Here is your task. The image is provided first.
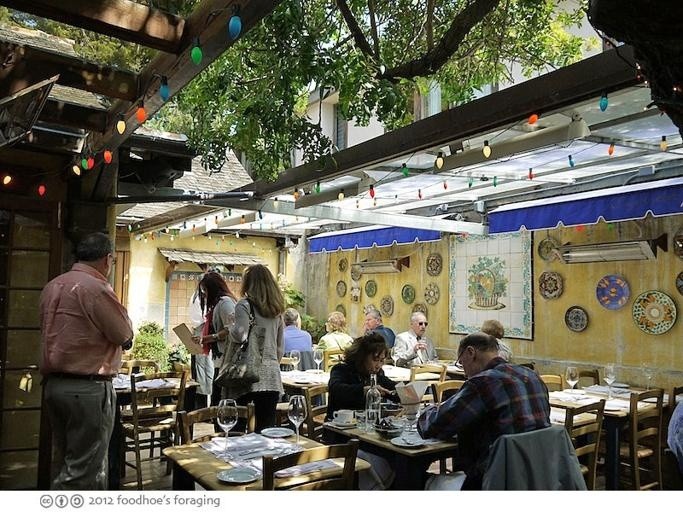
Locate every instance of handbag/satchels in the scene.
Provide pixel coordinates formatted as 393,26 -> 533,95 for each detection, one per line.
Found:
214,322 -> 266,385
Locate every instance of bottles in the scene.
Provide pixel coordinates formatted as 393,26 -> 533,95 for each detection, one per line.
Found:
366,374 -> 381,433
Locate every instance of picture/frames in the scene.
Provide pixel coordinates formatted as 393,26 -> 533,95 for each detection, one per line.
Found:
448,231 -> 535,341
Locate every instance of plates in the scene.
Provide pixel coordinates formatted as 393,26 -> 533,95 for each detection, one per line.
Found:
538,238 -> 559,261
596,274 -> 630,310
676,271 -> 683,295
351,267 -> 361,280
217,469 -> 260,483
391,437 -> 422,447
468,302 -> 505,309
336,304 -> 345,316
565,306 -> 588,332
351,288 -> 361,303
261,427 -> 294,438
632,290 -> 677,335
401,284 -> 415,304
336,281 -> 346,297
674,226 -> 683,258
605,407 -> 621,411
365,280 -> 377,297
538,272 -> 563,298
338,258 -> 348,272
564,389 -> 585,395
612,383 -> 629,387
332,422 -> 357,426
644,394 -> 680,402
295,381 -> 310,384
425,283 -> 440,304
381,296 -> 393,316
426,254 -> 441,275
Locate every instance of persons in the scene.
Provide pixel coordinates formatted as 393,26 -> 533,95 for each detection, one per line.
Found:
38,232 -> 134,490
220,264 -> 285,433
417,332 -> 552,490
283,308 -> 312,353
191,272 -> 238,406
482,320 -> 513,363
322,332 -> 402,490
363,308 -> 395,350
317,311 -> 354,372
667,399 -> 683,471
189,265 -> 230,397
391,311 -> 436,368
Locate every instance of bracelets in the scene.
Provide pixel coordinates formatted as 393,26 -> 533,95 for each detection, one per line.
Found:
212,334 -> 218,342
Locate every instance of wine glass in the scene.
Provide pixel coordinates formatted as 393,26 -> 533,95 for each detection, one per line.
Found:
313,348 -> 324,374
565,367 -> 578,394
288,395 -> 307,451
391,347 -> 399,368
290,350 -> 300,372
604,360 -> 617,400
216,399 -> 238,459
641,360 -> 654,391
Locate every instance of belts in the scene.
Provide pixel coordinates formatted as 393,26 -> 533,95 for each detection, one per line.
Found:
39,373 -> 112,383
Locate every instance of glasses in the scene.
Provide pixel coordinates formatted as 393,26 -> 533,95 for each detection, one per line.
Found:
454,346 -> 467,368
109,254 -> 117,265
418,321 -> 428,326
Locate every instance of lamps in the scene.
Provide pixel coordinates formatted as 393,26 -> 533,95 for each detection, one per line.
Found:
295,170 -> 378,209
180,218 -> 212,238
433,108 -> 592,176
551,234 -> 668,265
217,210 -> 261,229
351,256 -> 410,274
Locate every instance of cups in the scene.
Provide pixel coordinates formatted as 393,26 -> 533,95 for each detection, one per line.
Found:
333,409 -> 353,424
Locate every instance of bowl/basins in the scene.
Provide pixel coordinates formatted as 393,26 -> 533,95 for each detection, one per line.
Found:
380,403 -> 403,416
375,424 -> 403,438
